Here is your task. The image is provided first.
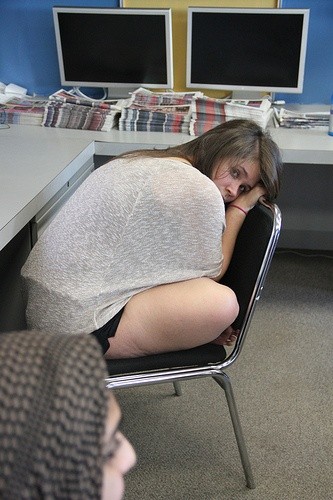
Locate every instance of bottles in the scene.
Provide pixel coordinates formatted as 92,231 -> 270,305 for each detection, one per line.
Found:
329,96 -> 333,136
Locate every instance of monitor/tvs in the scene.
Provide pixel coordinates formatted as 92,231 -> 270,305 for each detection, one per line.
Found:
52,7 -> 173,98
186,7 -> 310,100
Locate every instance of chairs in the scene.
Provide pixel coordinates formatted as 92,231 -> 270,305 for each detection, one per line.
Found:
106,197 -> 282,489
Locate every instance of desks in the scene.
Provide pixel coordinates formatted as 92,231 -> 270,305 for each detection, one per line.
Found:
0,122 -> 94,251
94,101 -> 333,253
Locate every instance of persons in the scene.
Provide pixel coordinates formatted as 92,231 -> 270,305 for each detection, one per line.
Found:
0,330 -> 137,500
21,118 -> 284,360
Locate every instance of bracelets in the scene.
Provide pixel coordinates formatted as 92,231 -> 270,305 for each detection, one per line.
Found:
228,203 -> 249,216
